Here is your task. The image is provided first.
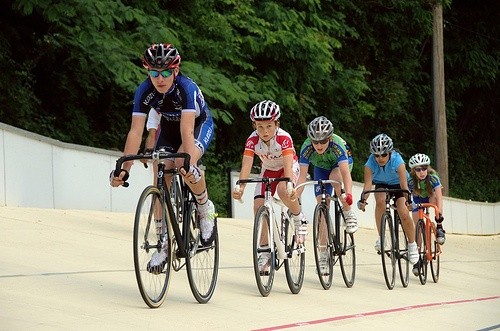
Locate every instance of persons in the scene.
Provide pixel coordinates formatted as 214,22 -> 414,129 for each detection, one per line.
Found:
288,117 -> 358,272
409,153 -> 445,275
233,100 -> 309,269
110,43 -> 214,267
358,133 -> 419,264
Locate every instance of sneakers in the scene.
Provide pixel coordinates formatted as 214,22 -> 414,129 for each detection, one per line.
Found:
407,242 -> 419,264
344,211 -> 358,233
294,215 -> 308,244
198,200 -> 215,246
146,234 -> 168,274
413,259 -> 422,274
436,229 -> 445,244
375,237 -> 387,251
317,254 -> 329,275
258,253 -> 272,272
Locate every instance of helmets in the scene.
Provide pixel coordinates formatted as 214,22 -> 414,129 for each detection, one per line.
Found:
142,43 -> 181,70
307,116 -> 334,141
249,100 -> 282,122
370,133 -> 393,154
409,153 -> 430,168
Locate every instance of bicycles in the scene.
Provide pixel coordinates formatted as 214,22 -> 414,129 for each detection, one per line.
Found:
360,188 -> 411,290
411,203 -> 443,285
114,148 -> 219,309
295,179 -> 357,291
234,177 -> 306,298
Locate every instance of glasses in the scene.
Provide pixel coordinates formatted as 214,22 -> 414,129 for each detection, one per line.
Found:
311,138 -> 330,144
415,168 -> 427,172
375,151 -> 388,157
147,67 -> 175,78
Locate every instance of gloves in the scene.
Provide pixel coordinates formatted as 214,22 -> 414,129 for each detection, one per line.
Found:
435,213 -> 444,222
